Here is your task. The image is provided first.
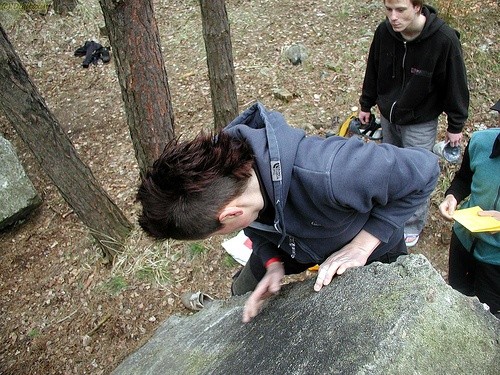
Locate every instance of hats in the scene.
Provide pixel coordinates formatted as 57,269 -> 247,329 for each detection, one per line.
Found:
490,98 -> 500,111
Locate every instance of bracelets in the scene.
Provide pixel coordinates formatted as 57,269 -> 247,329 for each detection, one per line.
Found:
266,257 -> 278,267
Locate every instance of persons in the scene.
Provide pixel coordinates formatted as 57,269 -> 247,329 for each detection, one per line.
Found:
135,104 -> 439,324
359,0 -> 469,246
439,99 -> 500,317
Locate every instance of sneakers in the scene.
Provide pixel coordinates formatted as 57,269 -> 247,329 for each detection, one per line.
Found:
181,291 -> 215,311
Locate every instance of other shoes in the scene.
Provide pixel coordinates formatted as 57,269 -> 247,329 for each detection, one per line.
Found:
349,113 -> 383,140
404,232 -> 419,246
